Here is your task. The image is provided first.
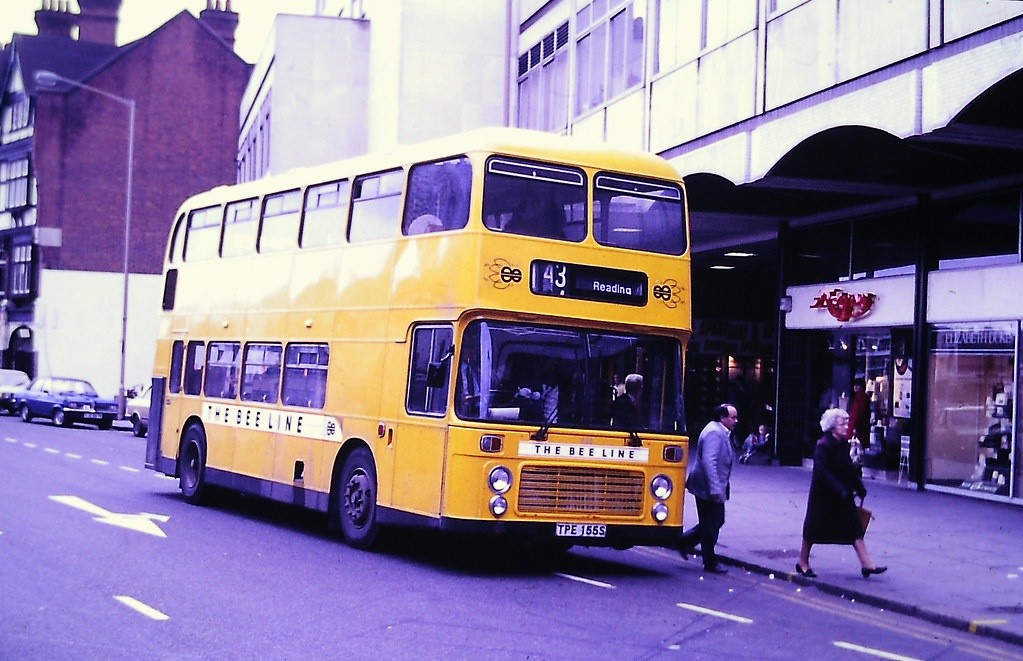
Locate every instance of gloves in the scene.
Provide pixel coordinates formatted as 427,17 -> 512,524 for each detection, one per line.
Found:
856,487 -> 866,499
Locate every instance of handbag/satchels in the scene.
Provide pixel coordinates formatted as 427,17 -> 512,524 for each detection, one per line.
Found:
853,493 -> 872,539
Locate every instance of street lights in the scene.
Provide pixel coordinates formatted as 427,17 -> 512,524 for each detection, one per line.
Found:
33,70 -> 137,423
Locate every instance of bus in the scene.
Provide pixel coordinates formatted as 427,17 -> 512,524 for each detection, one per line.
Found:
144,127 -> 693,563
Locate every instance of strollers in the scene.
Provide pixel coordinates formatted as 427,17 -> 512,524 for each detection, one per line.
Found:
737,433 -> 770,464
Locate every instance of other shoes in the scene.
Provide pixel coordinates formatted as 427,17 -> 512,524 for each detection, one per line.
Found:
703,562 -> 729,573
679,548 -> 688,560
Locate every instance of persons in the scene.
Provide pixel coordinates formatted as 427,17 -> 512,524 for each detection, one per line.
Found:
795,408 -> 887,580
844,378 -> 876,480
739,424 -> 771,465
678,402 -> 739,573
440,338 -> 648,429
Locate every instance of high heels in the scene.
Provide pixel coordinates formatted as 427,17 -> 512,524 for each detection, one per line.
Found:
796,563 -> 817,578
861,565 -> 887,578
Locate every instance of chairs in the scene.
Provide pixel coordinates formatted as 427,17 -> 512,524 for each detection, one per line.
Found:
747,433 -> 774,466
198,365 -> 237,399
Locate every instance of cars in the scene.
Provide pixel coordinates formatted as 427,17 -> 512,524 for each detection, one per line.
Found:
126,385 -> 154,438
0,368 -> 31,415
11,376 -> 120,431
